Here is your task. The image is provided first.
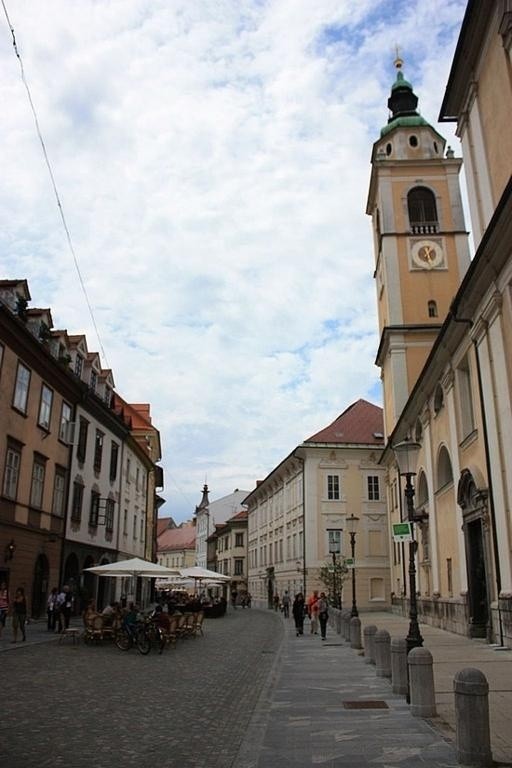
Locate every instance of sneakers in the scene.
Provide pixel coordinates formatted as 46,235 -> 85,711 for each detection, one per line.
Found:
296,628 -> 326,640
10,628 -> 69,643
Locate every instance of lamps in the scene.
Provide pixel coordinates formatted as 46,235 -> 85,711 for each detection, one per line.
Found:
3,535 -> 16,563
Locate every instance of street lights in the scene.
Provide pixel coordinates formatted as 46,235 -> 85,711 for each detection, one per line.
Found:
387,431 -> 427,706
327,537 -> 342,609
343,511 -> 361,617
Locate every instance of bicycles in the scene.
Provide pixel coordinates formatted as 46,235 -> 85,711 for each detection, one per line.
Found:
113,609 -> 153,655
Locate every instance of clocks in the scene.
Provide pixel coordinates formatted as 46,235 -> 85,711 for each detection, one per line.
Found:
406,236 -> 449,271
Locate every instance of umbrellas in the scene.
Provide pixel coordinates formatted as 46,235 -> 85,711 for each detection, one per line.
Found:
82,556 -> 232,611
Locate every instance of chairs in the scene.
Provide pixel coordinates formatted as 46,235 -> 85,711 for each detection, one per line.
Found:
61,596 -> 229,653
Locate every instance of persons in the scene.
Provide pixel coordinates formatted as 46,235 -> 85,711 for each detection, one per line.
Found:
273,590 -> 330,640
0,579 -> 227,646
232,588 -> 252,610
